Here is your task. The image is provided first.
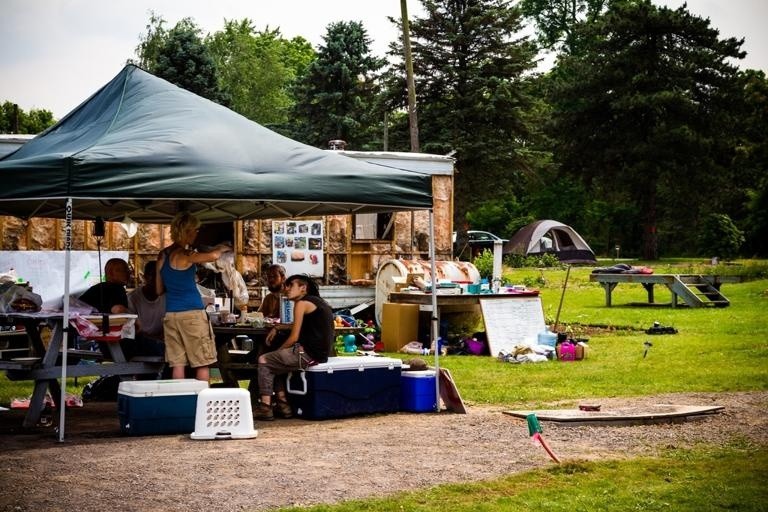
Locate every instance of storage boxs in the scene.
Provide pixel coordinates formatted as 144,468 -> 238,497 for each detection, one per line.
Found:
282,355 -> 441,422
115,377 -> 212,435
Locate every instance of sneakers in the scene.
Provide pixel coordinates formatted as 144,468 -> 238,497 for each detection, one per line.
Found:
252,405 -> 274,421
273,402 -> 294,419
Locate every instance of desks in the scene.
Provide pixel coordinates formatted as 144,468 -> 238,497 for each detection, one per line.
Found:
0,309 -> 140,430
387,283 -> 543,356
207,320 -> 365,403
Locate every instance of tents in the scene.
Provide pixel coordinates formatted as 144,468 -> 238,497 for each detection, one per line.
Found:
501,217 -> 599,269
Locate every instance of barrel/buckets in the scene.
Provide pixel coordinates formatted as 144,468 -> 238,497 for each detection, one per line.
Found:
374,261 -> 485,330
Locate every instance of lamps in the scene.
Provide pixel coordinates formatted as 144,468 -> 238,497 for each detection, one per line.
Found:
89,215 -> 108,244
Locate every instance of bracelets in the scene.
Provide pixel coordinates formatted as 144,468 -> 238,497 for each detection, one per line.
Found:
274,326 -> 279,332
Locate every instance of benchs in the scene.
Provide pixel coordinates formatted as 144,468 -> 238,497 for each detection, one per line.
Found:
0,347 -> 268,428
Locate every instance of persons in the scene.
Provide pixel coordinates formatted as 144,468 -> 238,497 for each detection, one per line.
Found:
156,211 -> 235,384
126,261 -> 165,354
75,257 -> 154,353
455,218 -> 472,260
243,271 -> 336,420
234,264 -> 288,385
